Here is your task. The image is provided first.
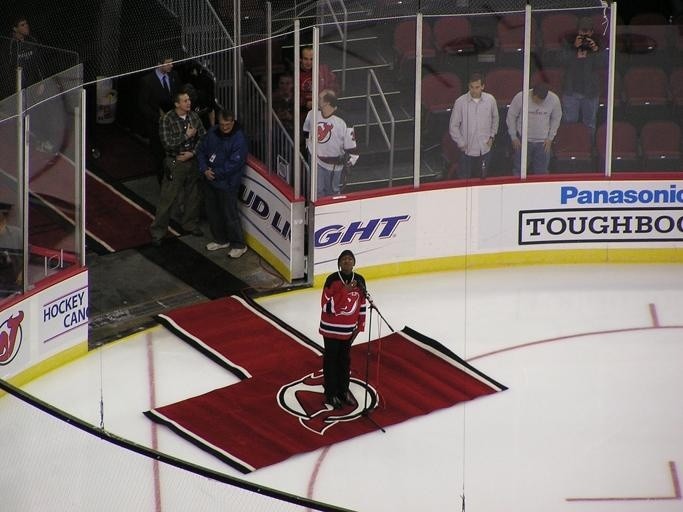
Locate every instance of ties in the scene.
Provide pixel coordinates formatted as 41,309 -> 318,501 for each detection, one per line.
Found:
161,74 -> 170,96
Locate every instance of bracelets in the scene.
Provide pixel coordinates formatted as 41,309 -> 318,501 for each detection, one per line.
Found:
489,137 -> 494,140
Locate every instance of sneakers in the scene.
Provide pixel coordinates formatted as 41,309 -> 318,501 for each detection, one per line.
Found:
324,394 -> 344,409
338,392 -> 356,406
205,240 -> 229,252
228,247 -> 248,258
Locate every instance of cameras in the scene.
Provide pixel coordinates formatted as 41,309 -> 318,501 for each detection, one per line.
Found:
578,35 -> 591,48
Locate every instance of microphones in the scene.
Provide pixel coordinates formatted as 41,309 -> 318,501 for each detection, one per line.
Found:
357,280 -> 366,291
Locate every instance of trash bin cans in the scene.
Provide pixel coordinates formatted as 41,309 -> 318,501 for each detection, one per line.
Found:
96,89 -> 118,125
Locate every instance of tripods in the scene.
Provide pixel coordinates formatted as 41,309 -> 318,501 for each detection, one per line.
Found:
344,291 -> 395,432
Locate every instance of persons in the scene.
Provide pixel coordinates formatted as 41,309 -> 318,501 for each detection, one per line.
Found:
504,83 -> 563,176
301,89 -> 360,199
447,70 -> 500,178
1,13 -> 43,97
196,106 -> 251,260
139,50 -> 182,188
557,16 -> 605,146
266,70 -> 308,138
291,44 -> 341,113
147,89 -> 206,249
317,247 -> 367,410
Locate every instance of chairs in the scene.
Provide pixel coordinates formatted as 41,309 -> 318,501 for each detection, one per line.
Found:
390,0 -> 683,179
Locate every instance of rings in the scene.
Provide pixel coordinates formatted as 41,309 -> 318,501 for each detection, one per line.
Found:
351,284 -> 353,286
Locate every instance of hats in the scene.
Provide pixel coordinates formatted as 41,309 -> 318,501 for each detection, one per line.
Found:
338,249 -> 356,264
